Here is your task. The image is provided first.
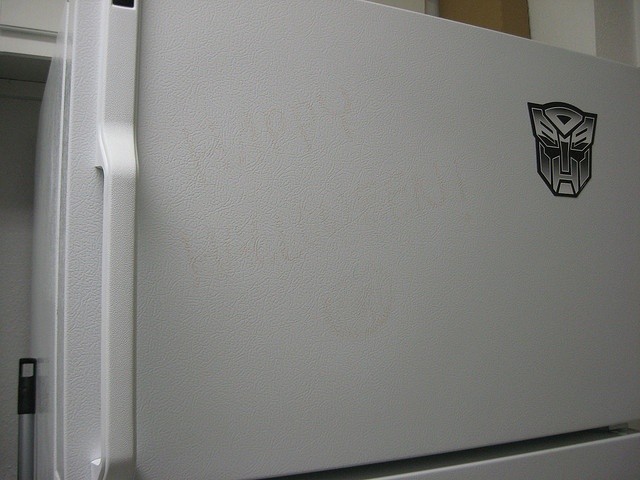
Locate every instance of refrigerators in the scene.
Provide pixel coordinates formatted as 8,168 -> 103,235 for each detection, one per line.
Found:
1,1 -> 640,479
